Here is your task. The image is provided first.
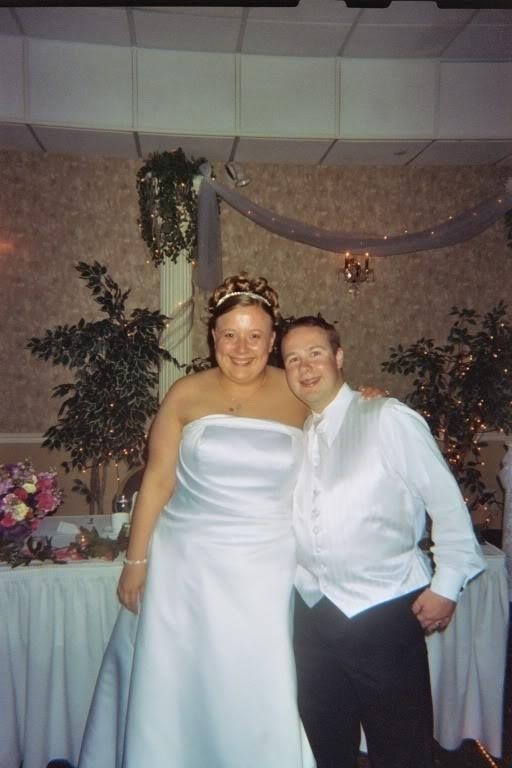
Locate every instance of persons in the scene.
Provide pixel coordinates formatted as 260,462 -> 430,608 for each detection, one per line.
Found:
74,276 -> 391,768
277,316 -> 486,767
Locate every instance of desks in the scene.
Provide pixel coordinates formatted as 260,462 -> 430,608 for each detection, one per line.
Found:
0,530 -> 510,768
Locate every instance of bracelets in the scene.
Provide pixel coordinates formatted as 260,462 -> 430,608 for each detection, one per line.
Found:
124,557 -> 147,565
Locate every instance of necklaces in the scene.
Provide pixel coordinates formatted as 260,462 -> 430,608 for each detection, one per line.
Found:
223,375 -> 267,411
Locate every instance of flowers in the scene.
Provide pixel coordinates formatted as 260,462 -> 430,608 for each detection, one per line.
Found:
0,457 -> 69,564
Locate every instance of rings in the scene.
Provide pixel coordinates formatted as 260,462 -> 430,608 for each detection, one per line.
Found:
385,390 -> 390,395
435,622 -> 441,628
